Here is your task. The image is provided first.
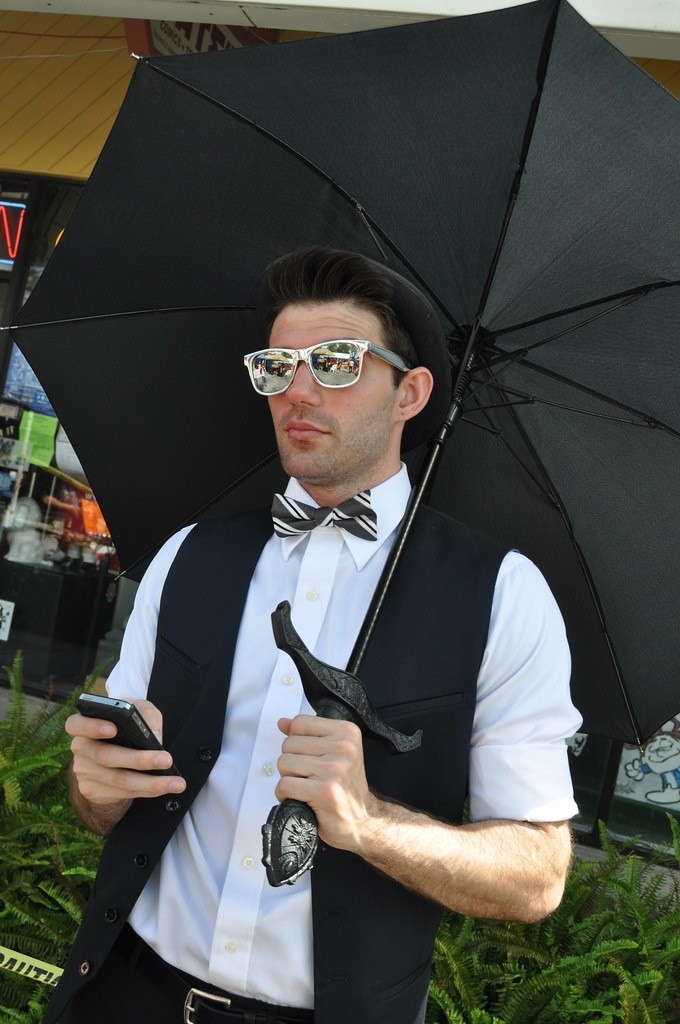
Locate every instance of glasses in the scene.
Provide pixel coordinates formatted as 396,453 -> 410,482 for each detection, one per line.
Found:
243,340 -> 412,396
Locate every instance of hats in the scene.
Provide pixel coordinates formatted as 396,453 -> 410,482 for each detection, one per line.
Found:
257,246 -> 451,454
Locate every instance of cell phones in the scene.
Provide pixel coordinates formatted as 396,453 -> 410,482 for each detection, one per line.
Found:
75,693 -> 180,780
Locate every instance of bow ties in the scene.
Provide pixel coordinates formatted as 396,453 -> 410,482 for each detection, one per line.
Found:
270,489 -> 376,543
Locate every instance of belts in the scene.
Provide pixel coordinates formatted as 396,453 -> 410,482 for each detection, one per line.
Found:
119,927 -> 312,1024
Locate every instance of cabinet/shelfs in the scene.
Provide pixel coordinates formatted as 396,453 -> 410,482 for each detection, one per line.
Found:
0,540 -> 121,702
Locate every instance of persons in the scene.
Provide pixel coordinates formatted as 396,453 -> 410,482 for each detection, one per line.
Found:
331,357 -> 357,374
40,246 -> 583,1024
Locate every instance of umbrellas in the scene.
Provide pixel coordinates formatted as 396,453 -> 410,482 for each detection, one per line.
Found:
255,354 -> 287,362
313,349 -> 333,355
1,0 -> 680,887
